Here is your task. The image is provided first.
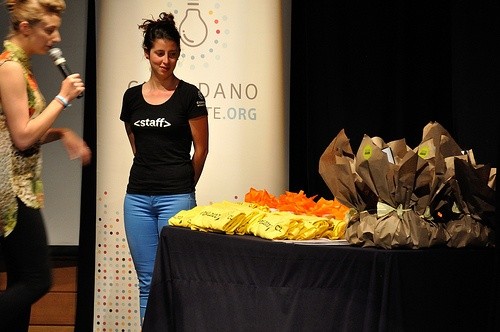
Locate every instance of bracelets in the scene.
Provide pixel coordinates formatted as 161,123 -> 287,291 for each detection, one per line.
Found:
55,95 -> 72,109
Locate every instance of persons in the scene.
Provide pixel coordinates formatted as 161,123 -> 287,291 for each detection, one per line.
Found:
0,0 -> 92,332
120,11 -> 209,327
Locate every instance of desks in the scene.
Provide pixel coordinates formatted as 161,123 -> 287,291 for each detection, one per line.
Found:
142,224 -> 500,332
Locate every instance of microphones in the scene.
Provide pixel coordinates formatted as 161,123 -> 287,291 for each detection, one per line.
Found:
48,47 -> 83,99
